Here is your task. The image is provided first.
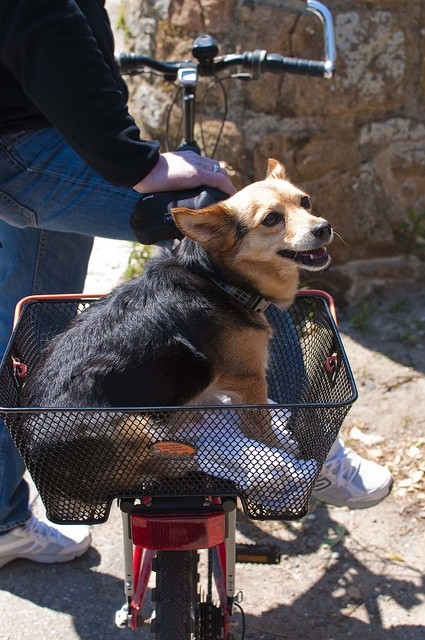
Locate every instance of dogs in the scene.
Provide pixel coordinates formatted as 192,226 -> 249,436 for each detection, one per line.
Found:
19,159 -> 333,506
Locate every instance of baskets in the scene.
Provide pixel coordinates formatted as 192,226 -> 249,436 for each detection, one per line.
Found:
0,289 -> 357,519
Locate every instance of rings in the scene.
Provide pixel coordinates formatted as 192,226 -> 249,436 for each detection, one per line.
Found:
213,162 -> 218,171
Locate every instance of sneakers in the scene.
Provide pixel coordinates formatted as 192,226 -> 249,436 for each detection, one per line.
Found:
311,435 -> 394,510
0,514 -> 92,571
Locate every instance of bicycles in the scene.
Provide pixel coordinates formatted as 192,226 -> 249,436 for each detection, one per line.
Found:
0,0 -> 356,640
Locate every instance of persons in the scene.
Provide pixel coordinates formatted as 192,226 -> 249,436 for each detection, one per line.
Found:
0,0 -> 393,571
